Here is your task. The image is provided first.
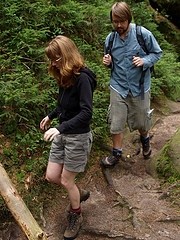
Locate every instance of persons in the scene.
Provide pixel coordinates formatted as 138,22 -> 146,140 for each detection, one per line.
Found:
40,35 -> 97,239
101,1 -> 163,169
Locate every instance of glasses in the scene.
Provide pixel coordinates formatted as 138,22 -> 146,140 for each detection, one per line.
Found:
112,19 -> 128,24
49,56 -> 62,63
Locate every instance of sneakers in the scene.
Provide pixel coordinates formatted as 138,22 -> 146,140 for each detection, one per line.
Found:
140,135 -> 151,158
101,147 -> 123,167
63,209 -> 83,238
65,187 -> 90,215
